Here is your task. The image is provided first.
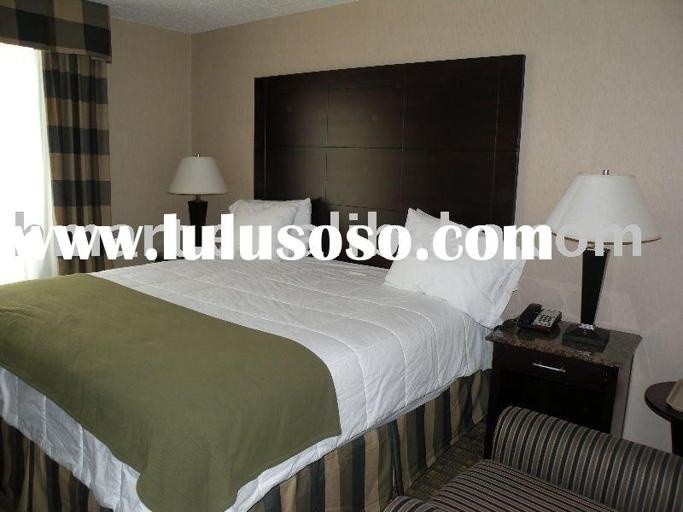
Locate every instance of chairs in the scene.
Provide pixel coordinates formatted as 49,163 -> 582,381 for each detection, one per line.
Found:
382,402 -> 683,511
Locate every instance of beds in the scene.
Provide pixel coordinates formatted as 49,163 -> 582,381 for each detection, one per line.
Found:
0,257 -> 494,512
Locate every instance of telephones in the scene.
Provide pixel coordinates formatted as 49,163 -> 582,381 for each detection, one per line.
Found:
516,303 -> 562,332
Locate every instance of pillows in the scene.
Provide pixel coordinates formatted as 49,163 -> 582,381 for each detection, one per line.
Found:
226,199 -> 311,261
380,205 -> 530,329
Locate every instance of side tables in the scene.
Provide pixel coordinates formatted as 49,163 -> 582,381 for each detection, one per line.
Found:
645,381 -> 683,455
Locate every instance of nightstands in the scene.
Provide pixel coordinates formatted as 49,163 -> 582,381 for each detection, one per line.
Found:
483,320 -> 645,467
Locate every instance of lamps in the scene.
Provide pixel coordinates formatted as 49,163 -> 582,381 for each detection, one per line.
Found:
168,153 -> 230,247
539,166 -> 660,352
